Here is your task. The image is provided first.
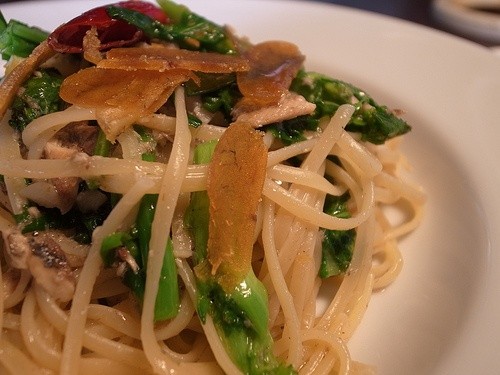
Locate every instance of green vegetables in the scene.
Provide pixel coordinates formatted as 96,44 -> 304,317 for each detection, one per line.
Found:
0,2 -> 415,375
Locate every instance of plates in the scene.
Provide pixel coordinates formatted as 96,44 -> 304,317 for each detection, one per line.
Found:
0,0 -> 499,373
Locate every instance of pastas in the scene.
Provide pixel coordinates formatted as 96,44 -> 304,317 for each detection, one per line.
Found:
1,87 -> 431,375
